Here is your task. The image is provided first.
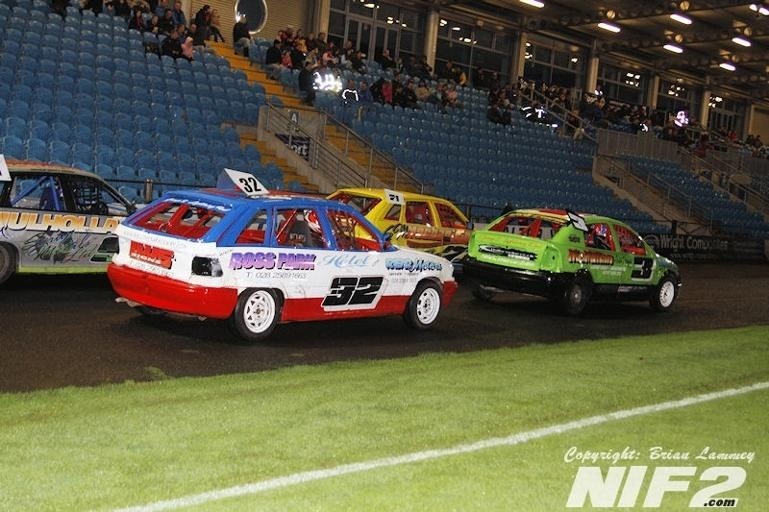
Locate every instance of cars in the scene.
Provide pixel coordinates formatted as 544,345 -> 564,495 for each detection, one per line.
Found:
106,191 -> 458,342
326,188 -> 476,280
0,160 -> 141,285
463,209 -> 681,317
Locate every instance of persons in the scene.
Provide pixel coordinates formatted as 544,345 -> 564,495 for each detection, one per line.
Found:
232,17 -> 467,114
472,71 -> 768,157
74,1 -> 226,63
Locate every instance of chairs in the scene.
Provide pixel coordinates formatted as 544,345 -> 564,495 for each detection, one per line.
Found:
237,1 -> 767,232
288,221 -> 314,247
0,1 -> 324,236
